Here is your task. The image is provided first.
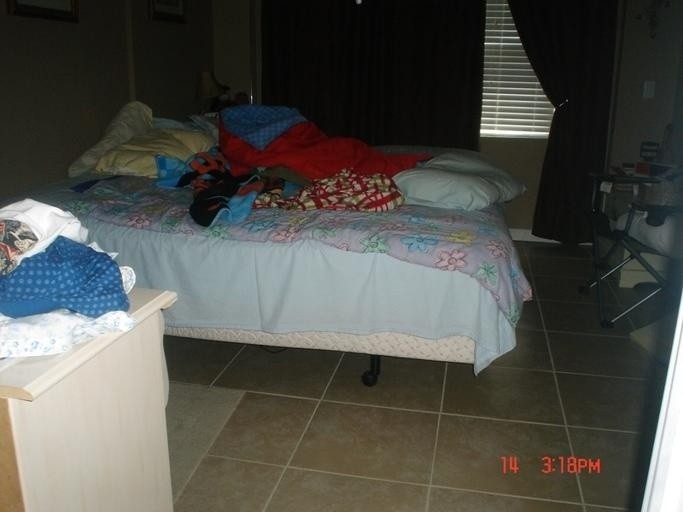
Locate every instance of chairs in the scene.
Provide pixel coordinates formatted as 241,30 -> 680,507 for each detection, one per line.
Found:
577,176 -> 682,328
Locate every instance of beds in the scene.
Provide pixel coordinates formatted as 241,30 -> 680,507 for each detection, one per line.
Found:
0,175 -> 536,389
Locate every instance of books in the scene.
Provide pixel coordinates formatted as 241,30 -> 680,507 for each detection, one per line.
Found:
604,152 -> 683,208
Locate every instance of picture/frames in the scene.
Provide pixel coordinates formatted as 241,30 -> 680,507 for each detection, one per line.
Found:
147,0 -> 187,23
6,0 -> 81,25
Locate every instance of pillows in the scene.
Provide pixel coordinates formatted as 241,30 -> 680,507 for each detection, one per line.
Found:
391,152 -> 528,212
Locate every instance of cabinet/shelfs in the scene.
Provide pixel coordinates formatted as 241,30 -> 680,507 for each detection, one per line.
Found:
0,286 -> 179,512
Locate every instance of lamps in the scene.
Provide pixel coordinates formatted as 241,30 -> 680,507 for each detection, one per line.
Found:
195,71 -> 223,112
